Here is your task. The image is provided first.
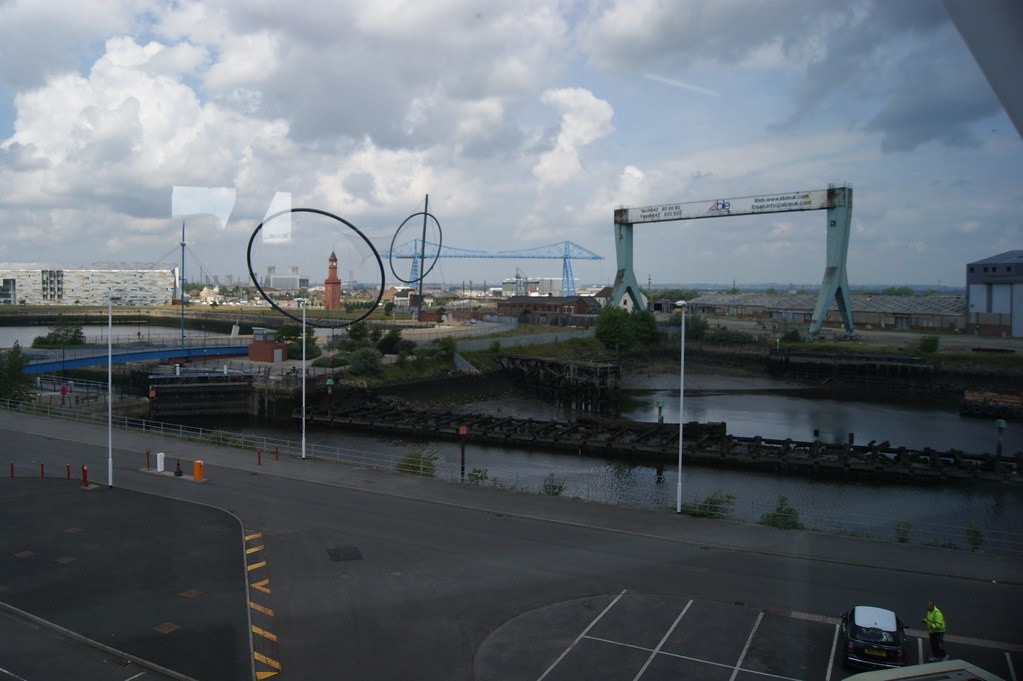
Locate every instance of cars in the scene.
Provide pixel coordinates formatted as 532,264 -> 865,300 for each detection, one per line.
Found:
839,605 -> 910,673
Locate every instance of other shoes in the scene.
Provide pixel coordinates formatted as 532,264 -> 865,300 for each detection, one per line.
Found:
928,656 -> 938,661
940,654 -> 949,661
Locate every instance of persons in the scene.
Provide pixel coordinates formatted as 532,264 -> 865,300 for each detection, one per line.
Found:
291,365 -> 299,378
60,383 -> 70,403
921,599 -> 951,663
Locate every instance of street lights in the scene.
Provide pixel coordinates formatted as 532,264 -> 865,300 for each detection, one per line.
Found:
108,294 -> 122,489
675,301 -> 687,514
293,297 -> 306,459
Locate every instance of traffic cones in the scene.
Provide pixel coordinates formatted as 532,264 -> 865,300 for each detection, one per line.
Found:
174,459 -> 183,477
